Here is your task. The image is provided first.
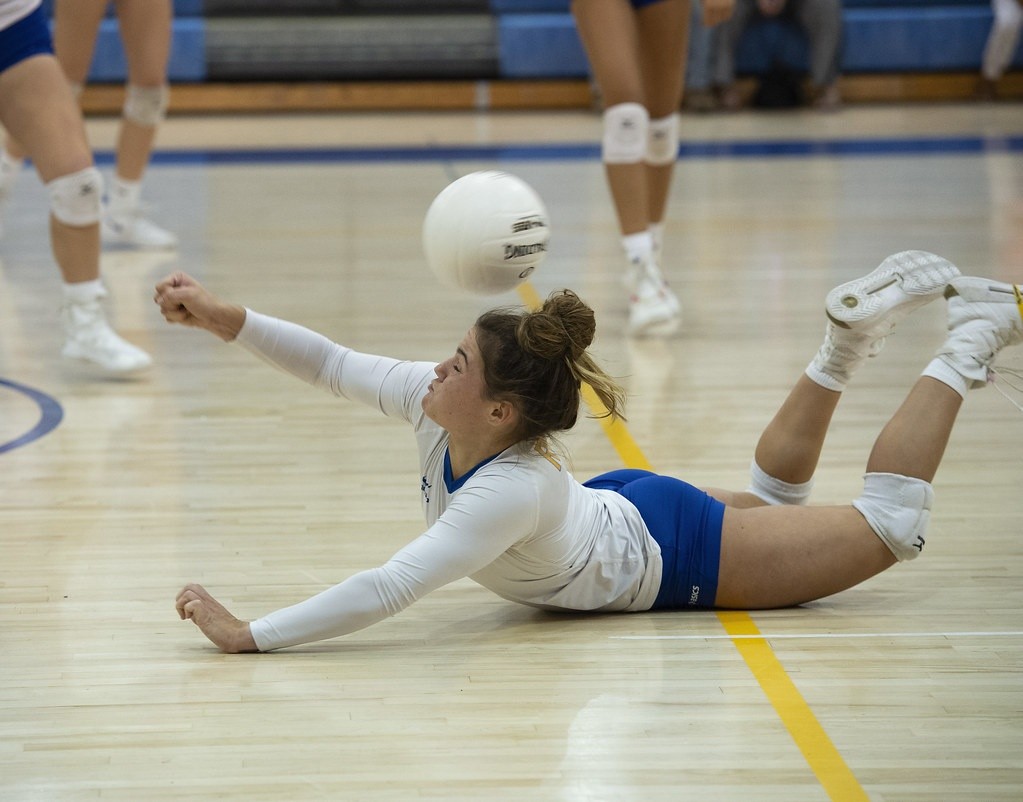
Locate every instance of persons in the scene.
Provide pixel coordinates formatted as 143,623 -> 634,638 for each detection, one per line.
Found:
155,249 -> 1022,653
0,0 -> 153,372
979,0 -> 1023,81
570,0 -> 734,326
0,0 -> 174,247
690,0 -> 839,111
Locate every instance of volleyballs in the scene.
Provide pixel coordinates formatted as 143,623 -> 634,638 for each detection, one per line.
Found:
422,168 -> 552,298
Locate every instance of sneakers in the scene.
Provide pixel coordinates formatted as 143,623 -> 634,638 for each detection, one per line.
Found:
625,253 -> 682,337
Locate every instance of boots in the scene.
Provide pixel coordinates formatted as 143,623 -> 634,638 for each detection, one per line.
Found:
99,194 -> 174,249
936,277 -> 1023,410
62,296 -> 151,374
813,250 -> 965,384
